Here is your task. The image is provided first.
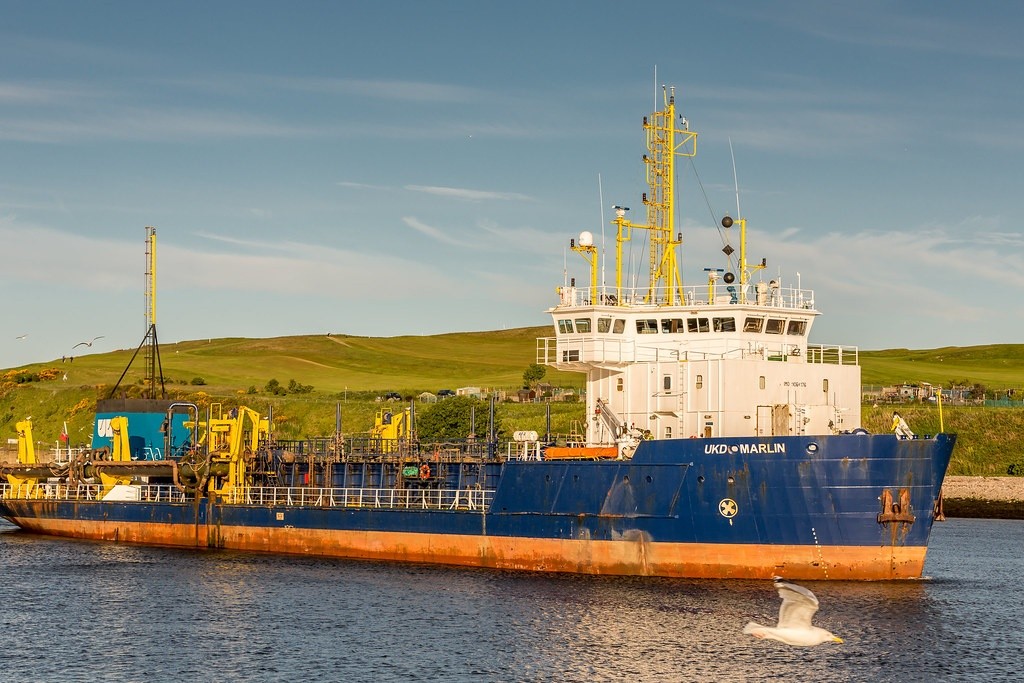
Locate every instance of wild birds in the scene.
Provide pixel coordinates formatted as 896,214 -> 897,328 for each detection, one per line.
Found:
63,336 -> 105,380
16,335 -> 28,340
741,576 -> 844,647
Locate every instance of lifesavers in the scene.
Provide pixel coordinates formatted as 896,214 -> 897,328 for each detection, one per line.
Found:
419,465 -> 430,480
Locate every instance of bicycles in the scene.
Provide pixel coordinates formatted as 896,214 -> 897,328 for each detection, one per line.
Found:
1007,464 -> 1024,477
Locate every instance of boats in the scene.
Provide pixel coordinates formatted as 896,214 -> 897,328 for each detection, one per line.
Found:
0,66 -> 958,581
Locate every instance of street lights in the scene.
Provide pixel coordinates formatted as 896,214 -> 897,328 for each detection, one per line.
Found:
345,385 -> 348,403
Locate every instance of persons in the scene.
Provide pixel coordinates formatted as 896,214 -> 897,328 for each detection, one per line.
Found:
699,433 -> 703,437
63,356 -> 66,363
70,356 -> 73,362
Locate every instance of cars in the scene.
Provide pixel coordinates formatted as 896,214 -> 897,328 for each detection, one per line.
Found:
386,393 -> 402,401
438,389 -> 455,397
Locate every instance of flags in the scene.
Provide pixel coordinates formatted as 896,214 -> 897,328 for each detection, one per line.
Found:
60,433 -> 67,441
